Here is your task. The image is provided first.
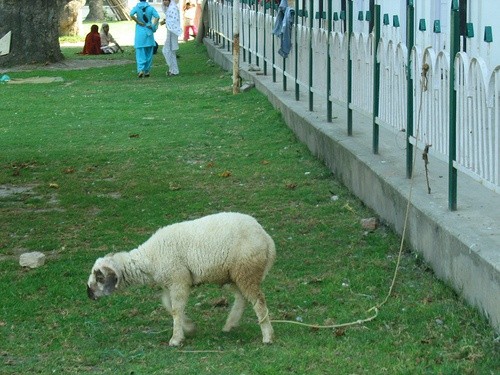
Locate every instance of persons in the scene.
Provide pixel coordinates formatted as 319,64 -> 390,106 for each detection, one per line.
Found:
82,23 -> 124,55
160,0 -> 182,75
130,0 -> 160,78
184,2 -> 198,41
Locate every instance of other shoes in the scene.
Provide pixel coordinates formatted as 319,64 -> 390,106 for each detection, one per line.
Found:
145,71 -> 150,77
166,72 -> 177,76
138,71 -> 143,78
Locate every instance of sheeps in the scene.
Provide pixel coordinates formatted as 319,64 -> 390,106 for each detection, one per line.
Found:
87,211 -> 276,348
